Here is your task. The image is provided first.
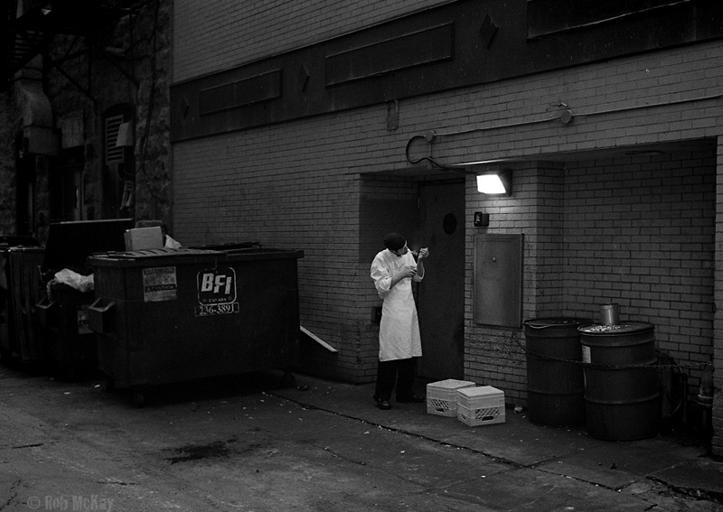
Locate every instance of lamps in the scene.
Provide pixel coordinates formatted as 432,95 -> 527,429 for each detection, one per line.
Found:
476,168 -> 512,196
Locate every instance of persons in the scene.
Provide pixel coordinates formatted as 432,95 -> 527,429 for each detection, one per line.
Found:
368,233 -> 430,411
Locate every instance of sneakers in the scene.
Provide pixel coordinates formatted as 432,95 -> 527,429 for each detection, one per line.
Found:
373,396 -> 391,409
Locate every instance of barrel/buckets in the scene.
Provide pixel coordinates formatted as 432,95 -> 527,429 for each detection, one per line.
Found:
576,318 -> 663,442
523,317 -> 593,426
